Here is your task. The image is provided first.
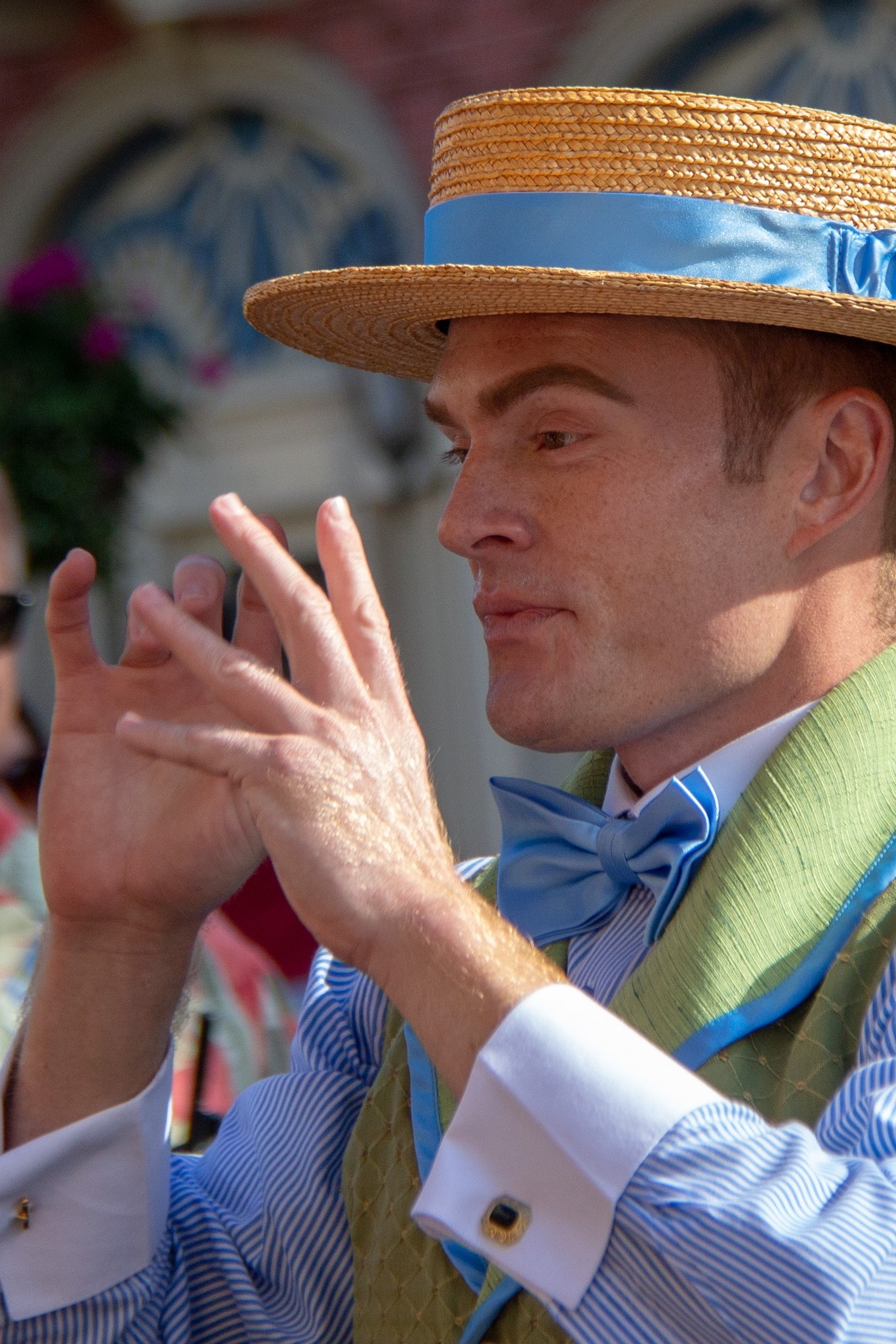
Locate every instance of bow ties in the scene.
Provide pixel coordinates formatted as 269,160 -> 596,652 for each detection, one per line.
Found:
491,768 -> 719,953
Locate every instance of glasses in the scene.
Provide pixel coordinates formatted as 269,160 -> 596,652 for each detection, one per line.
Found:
0,593 -> 29,652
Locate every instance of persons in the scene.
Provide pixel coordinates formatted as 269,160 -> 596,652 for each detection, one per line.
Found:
0,294 -> 297,1151
0,86 -> 895,1344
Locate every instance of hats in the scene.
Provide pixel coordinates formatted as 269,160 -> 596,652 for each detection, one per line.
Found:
241,83 -> 896,381
